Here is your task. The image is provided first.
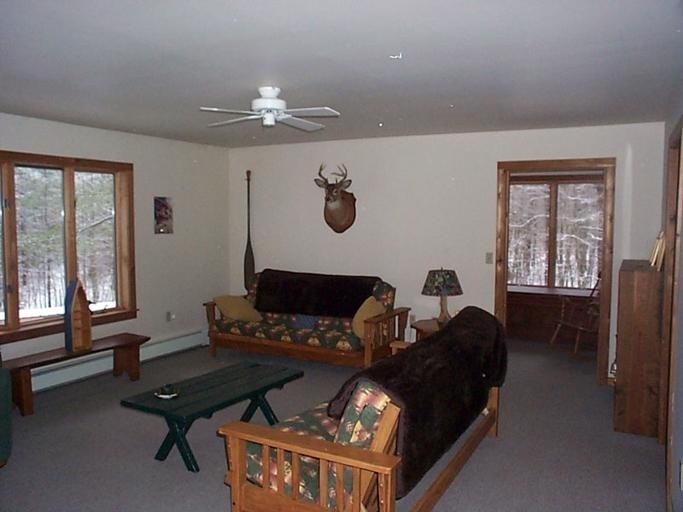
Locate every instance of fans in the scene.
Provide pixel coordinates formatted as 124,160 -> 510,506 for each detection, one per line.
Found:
200,85 -> 340,132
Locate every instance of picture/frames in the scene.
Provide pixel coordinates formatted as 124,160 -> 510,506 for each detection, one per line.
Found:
154,196 -> 173,234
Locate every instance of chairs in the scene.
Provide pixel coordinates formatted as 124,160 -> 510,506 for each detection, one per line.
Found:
549,272 -> 603,354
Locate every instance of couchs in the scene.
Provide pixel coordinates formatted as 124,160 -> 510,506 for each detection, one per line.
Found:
216,305 -> 508,512
206,271 -> 411,371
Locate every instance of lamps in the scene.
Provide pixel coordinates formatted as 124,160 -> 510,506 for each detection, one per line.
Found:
252,86 -> 286,127
421,267 -> 463,324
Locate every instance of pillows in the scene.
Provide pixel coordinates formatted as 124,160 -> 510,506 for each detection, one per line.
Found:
351,297 -> 389,339
209,295 -> 263,321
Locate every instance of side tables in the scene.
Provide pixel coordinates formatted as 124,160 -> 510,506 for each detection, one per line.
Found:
410,318 -> 454,341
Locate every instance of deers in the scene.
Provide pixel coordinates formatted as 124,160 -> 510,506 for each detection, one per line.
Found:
313,163 -> 358,233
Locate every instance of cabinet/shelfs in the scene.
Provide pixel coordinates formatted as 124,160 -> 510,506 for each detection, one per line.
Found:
613,260 -> 663,437
